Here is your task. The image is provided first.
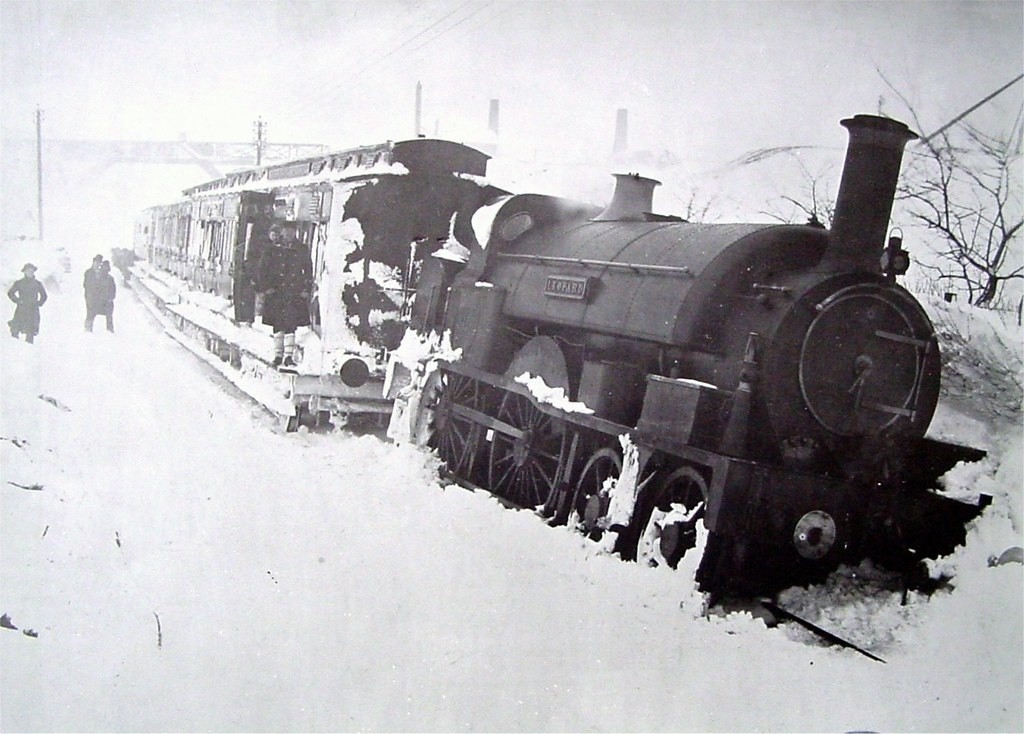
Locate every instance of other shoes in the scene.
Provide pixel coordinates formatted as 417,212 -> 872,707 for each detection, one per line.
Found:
284,356 -> 297,368
269,356 -> 282,366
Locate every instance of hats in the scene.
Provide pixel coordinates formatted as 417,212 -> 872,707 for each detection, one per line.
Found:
100,260 -> 110,267
93,254 -> 103,262
21,263 -> 38,272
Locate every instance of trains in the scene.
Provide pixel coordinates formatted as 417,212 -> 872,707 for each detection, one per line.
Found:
126,114 -> 994,663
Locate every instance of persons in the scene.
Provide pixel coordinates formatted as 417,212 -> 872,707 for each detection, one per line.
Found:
83,254 -> 116,332
250,221 -> 313,367
7,263 -> 48,344
